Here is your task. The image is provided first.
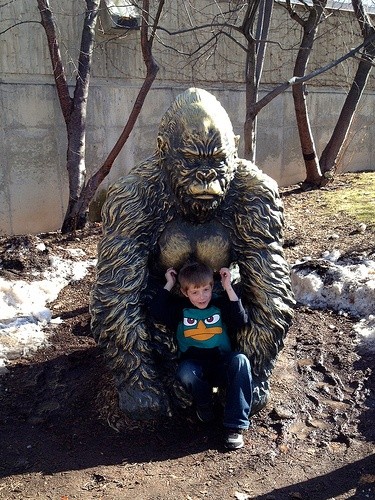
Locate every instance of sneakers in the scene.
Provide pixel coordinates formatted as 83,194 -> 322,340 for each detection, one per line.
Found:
225,428 -> 245,450
195,398 -> 215,422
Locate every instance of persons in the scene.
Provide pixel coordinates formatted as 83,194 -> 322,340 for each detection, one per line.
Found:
148,262 -> 254,449
